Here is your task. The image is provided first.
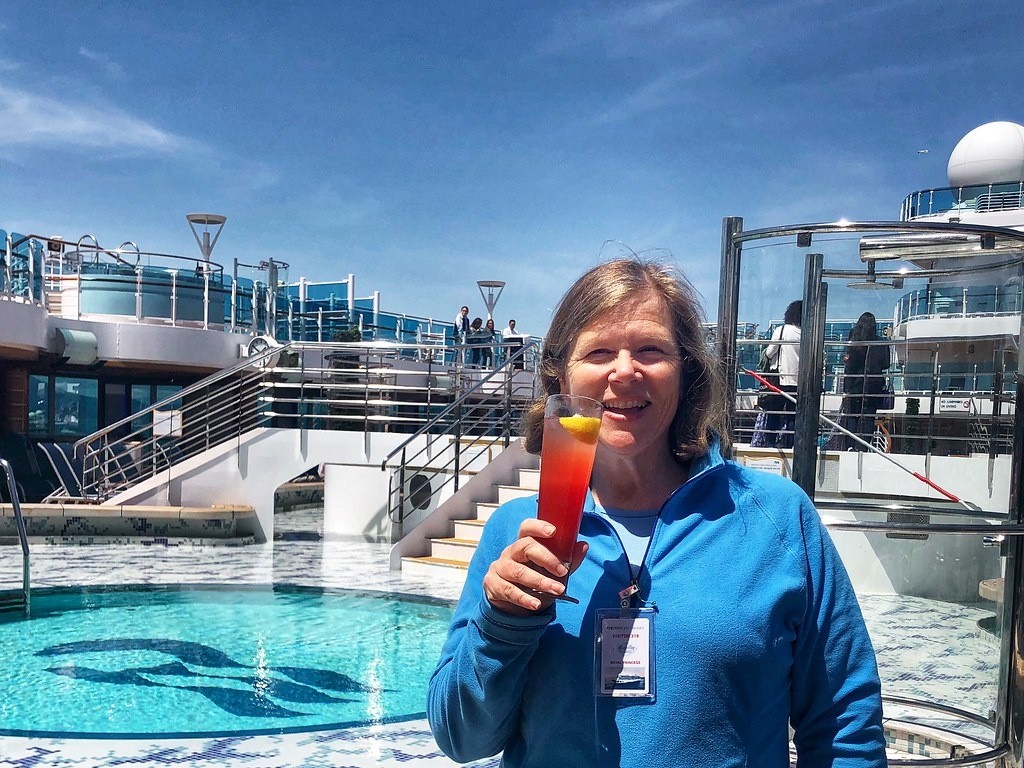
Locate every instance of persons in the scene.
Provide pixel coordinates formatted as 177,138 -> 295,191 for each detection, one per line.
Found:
762,300 -> 802,448
843,312 -> 890,452
425,260 -> 887,768
453,306 -> 469,343
467,318 -> 483,368
503,320 -> 516,335
482,318 -> 495,369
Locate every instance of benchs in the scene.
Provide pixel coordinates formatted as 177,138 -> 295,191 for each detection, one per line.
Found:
49,496 -> 104,504
0,503 -> 255,538
276,482 -> 325,503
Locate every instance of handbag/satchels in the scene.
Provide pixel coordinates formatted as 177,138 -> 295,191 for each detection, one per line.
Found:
758,368 -> 781,412
878,388 -> 894,410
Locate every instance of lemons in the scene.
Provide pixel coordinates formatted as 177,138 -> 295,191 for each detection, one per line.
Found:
559,415 -> 601,446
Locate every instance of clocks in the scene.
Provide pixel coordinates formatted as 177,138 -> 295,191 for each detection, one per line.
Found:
247,335 -> 279,369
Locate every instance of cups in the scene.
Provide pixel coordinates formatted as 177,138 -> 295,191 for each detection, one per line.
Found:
530,395 -> 604,602
